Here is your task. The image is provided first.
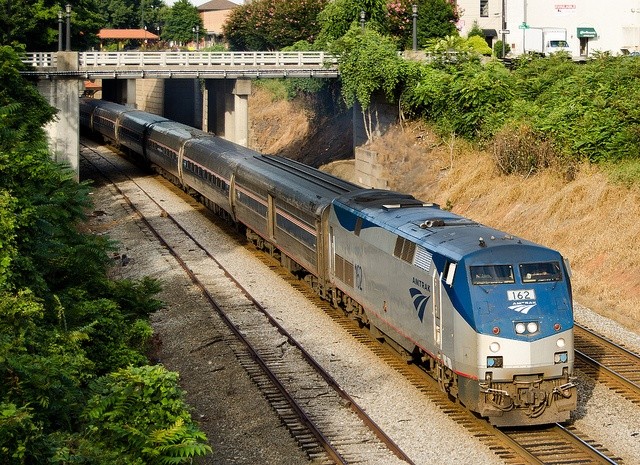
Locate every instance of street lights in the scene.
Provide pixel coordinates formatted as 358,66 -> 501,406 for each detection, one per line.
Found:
145,26 -> 147,50
66,5 -> 71,52
360,11 -> 365,48
58,10 -> 62,51
412,4 -> 417,50
157,27 -> 160,40
195,26 -> 200,52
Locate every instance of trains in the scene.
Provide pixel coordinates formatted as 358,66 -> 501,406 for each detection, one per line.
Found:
80,95 -> 578,429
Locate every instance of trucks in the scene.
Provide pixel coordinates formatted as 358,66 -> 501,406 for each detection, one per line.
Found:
524,27 -> 572,59
